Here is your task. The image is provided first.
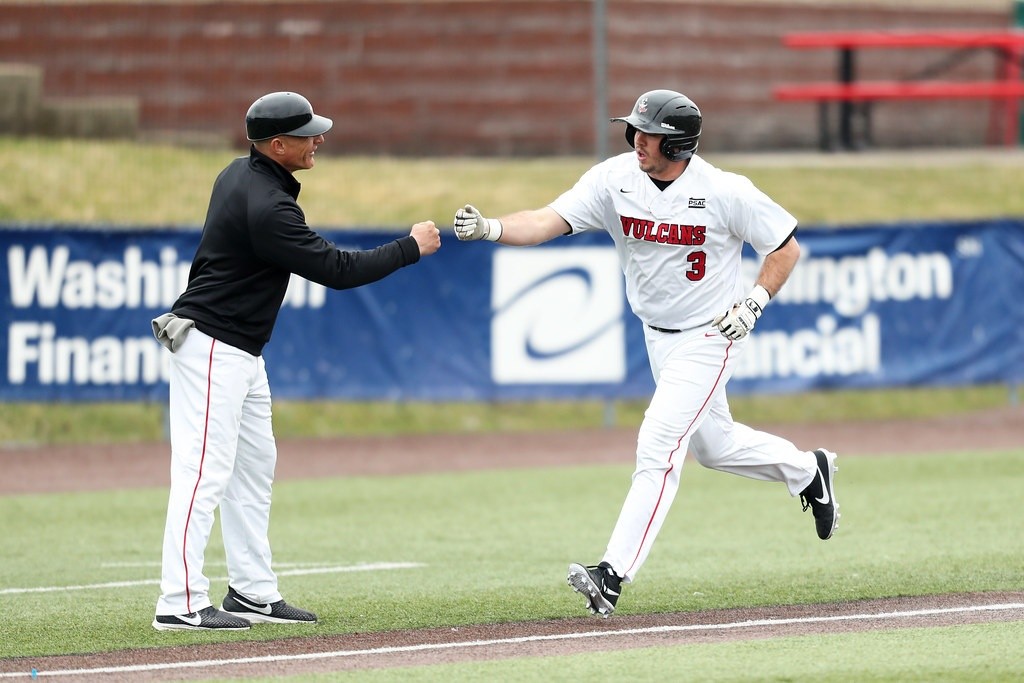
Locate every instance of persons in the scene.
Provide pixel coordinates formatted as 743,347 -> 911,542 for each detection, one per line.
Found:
152,91 -> 443,632
452,87 -> 840,614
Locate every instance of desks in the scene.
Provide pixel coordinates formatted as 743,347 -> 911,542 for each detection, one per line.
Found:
779,30 -> 1024,151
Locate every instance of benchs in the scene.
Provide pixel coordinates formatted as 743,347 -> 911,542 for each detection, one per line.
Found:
771,79 -> 1024,97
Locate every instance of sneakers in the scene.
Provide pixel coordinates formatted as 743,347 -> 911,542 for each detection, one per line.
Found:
219,585 -> 317,623
799,447 -> 841,541
151,606 -> 251,631
566,561 -> 622,620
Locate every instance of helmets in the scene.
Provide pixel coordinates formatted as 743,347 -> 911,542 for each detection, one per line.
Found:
610,89 -> 703,162
245,91 -> 334,141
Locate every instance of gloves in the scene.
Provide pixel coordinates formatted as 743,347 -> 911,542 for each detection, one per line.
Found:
151,313 -> 196,353
454,204 -> 490,242
711,297 -> 762,341
